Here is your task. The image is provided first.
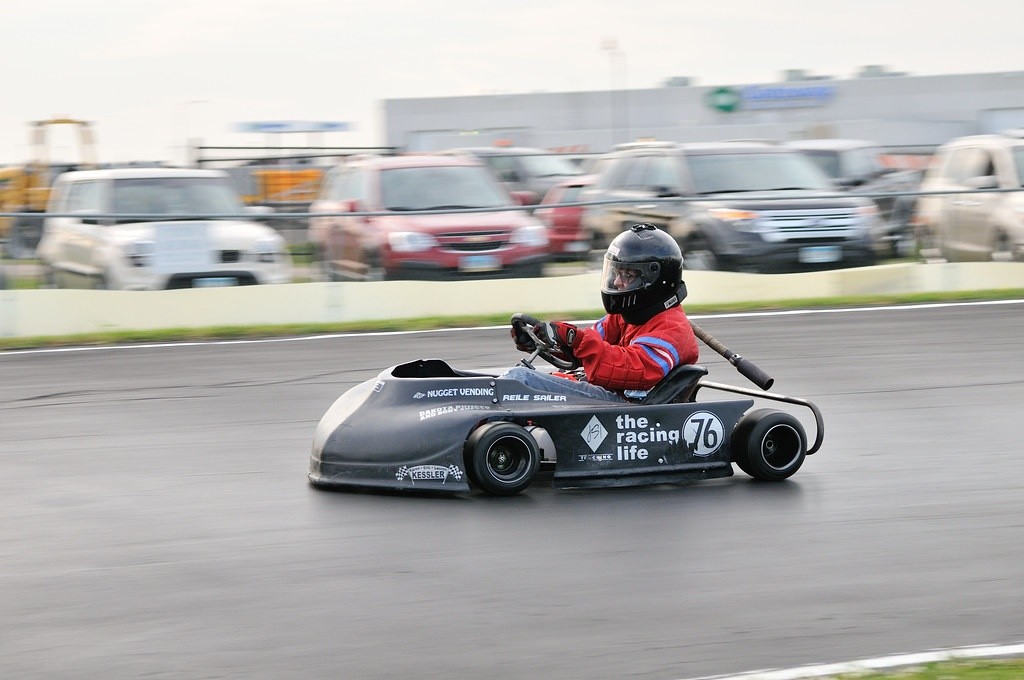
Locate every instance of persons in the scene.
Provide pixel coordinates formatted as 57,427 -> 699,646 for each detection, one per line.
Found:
510,223 -> 699,404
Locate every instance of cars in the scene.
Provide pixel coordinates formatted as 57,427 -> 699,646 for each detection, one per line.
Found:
302,153 -> 548,282
581,145 -> 882,272
446,135 -> 923,262
36,168 -> 296,291
910,131 -> 1024,262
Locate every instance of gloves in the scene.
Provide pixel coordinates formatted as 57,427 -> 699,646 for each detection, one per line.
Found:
510,327 -> 537,354
532,320 -> 579,351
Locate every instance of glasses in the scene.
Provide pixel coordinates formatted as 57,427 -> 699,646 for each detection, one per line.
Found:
608,266 -> 639,287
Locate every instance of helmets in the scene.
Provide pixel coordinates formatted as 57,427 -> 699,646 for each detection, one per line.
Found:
599,221 -> 687,315
